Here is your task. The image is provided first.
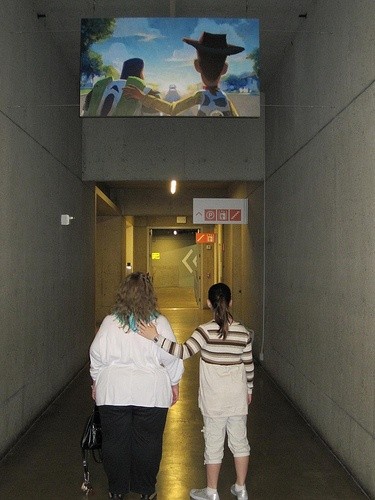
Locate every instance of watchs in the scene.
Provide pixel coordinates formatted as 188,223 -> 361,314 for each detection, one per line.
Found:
153,335 -> 160,343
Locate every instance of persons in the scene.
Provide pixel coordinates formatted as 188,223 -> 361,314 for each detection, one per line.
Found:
134,283 -> 255,500
90,272 -> 183,500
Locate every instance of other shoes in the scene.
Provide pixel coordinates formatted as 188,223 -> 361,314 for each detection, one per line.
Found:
139,493 -> 157,500
109,492 -> 123,500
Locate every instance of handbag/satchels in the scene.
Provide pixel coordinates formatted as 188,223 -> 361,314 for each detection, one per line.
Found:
80,400 -> 105,450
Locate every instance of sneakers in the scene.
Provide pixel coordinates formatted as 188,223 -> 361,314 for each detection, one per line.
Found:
230,483 -> 248,500
190,488 -> 219,500
